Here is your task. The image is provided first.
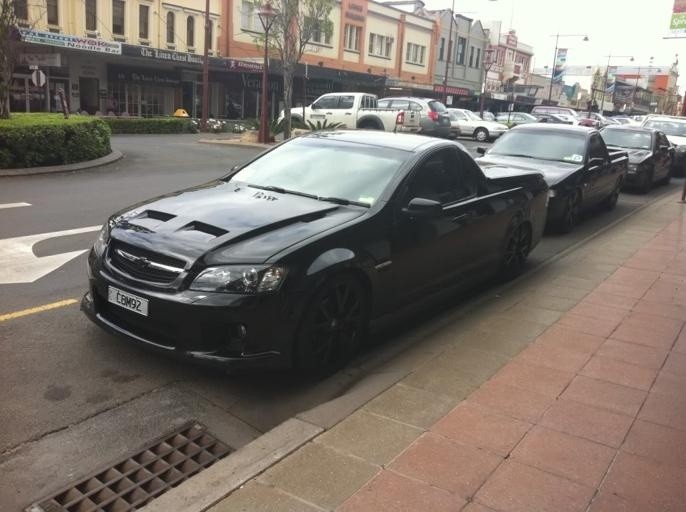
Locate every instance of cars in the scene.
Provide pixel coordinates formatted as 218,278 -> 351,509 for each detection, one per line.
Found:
591,125 -> 676,194
447,108 -> 508,143
79,128 -> 551,383
498,104 -> 686,175
379,96 -> 453,138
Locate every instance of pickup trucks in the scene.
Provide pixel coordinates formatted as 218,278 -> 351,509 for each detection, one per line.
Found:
225,94 -> 241,114
276,92 -> 422,133
476,123 -> 630,233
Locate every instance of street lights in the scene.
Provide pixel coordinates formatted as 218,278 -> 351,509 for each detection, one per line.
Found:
258,0 -> 278,143
547,33 -> 590,107
632,67 -> 663,103
587,84 -> 599,119
599,55 -> 635,116
479,44 -> 496,121
